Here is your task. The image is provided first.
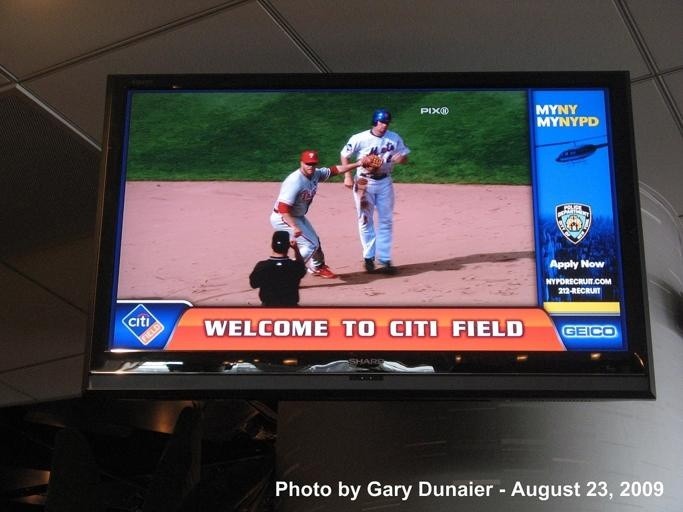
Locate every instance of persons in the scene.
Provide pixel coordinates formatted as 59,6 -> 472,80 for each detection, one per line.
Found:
249,230 -> 306,309
271,152 -> 371,279
338,106 -> 408,272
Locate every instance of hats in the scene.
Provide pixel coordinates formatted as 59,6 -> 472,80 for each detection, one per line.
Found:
271,230 -> 291,246
301,150 -> 318,164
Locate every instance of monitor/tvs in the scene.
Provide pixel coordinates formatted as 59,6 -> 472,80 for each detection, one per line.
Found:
81,71 -> 657,400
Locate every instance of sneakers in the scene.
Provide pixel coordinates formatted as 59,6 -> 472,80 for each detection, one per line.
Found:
311,260 -> 397,278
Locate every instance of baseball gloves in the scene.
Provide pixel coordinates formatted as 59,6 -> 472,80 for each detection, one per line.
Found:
362,155 -> 384,171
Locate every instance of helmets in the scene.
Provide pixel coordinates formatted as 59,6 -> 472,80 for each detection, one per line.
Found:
372,109 -> 392,128
